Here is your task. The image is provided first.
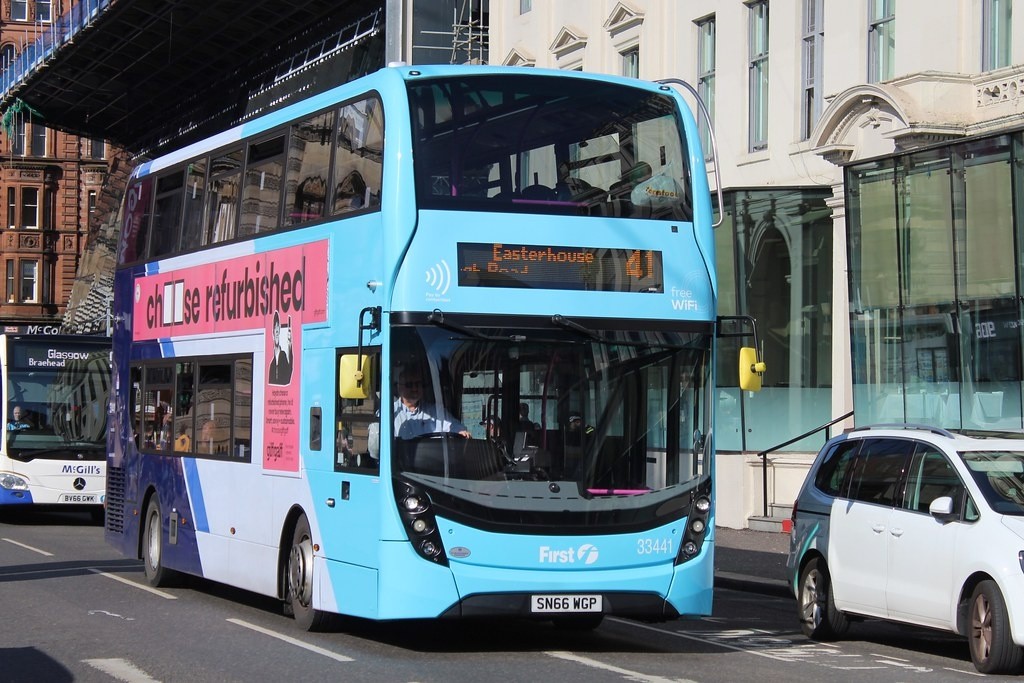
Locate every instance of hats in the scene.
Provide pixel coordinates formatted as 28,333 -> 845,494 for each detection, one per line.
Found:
565,411 -> 581,426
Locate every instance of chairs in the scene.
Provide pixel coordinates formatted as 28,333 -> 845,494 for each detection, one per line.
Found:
491,178 -> 592,216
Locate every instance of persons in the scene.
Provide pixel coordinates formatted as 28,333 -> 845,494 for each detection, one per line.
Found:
368,372 -> 471,442
7,406 -> 30,430
479,404 -> 595,442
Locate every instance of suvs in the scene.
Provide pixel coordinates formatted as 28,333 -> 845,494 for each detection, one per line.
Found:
786,424 -> 1024,676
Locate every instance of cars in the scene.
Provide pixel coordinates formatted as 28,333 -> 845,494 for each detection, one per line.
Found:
0,333 -> 113,527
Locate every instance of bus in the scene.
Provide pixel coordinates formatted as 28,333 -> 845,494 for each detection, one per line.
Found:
103,64 -> 768,632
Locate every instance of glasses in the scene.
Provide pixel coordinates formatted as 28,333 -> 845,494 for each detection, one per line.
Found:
398,381 -> 423,388
